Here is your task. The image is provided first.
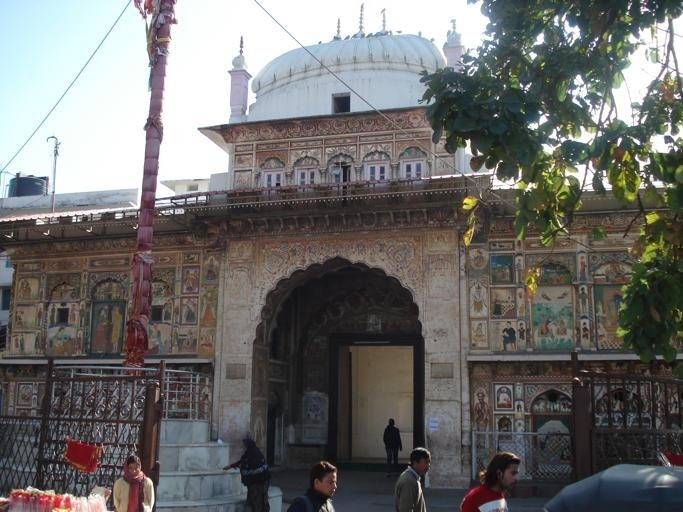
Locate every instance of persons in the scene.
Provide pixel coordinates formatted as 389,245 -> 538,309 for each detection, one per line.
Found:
394,447 -> 431,512
112,455 -> 155,512
287,461 -> 337,512
383,418 -> 402,467
459,452 -> 521,512
222,434 -> 271,512
471,321 -> 526,433
